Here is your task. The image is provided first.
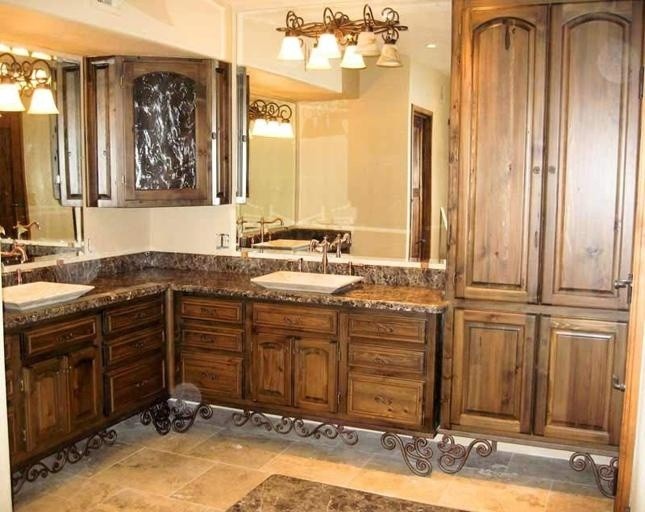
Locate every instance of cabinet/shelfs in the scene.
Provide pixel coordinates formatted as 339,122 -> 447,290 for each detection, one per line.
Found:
341,306 -> 434,438
170,288 -> 246,410
438,302 -> 630,451
50,59 -> 85,211
19,311 -> 106,458
5,326 -> 26,476
106,293 -> 170,426
449,0 -> 644,303
246,296 -> 341,421
81,56 -> 237,209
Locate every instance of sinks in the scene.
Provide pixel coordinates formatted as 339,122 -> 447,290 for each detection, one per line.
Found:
3,281 -> 95,311
248,271 -> 365,294
251,238 -> 311,248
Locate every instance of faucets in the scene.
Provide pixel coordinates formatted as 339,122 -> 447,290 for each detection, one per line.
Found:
257,215 -> 285,242
330,231 -> 351,257
12,220 -> 42,240
310,236 -> 332,274
2,246 -> 29,264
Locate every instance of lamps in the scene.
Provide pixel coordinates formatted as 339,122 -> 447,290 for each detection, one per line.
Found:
247,93 -> 302,139
273,2 -> 409,72
1,44 -> 62,117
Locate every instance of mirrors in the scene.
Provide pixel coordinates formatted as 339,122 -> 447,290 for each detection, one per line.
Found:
237,12 -> 451,265
1,42 -> 84,267
